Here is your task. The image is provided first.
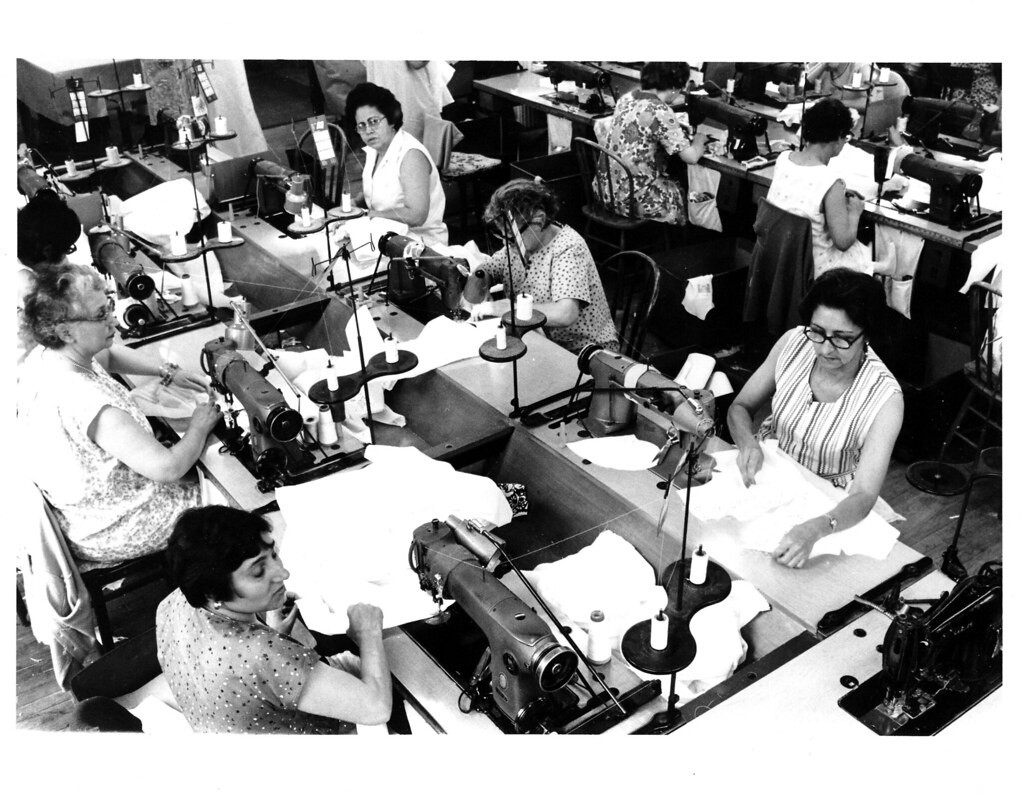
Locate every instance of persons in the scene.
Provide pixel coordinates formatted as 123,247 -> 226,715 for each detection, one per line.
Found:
362,60 -> 456,141
726,268 -> 905,569
16,266 -> 221,563
464,174 -> 620,356
765,99 -> 878,275
591,62 -> 721,226
17,190 -> 113,358
151,507 -> 394,733
345,83 -> 449,245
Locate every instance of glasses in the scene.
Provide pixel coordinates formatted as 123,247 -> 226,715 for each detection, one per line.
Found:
356,114 -> 386,131
840,130 -> 855,143
64,296 -> 116,323
803,321 -> 865,350
491,219 -> 532,246
56,243 -> 77,256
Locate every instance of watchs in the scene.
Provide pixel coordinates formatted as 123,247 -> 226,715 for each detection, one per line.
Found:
825,512 -> 837,533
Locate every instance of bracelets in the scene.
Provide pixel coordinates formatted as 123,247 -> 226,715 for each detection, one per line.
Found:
159,361 -> 180,387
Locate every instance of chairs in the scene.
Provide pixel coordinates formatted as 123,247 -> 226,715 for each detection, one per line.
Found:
16,122 -> 1002,733
